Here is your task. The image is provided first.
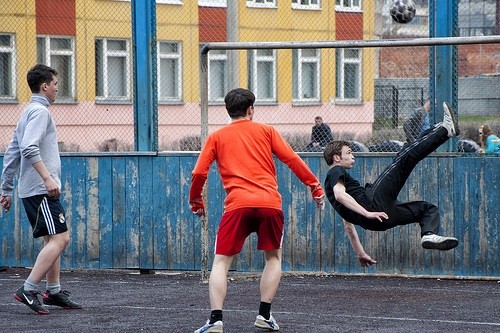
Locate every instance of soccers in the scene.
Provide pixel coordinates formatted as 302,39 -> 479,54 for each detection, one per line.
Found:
389,0 -> 417,24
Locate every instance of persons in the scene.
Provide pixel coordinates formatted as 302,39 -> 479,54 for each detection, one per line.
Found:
307,116 -> 334,152
403,98 -> 436,143
189,87 -> 326,333
324,99 -> 461,268
480,124 -> 500,154
0,64 -> 84,314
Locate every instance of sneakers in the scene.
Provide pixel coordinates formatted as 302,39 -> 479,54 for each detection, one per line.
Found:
13,285 -> 50,315
42,289 -> 82,309
433,102 -> 460,137
194,320 -> 225,333
421,233 -> 460,251
252,312 -> 280,330
